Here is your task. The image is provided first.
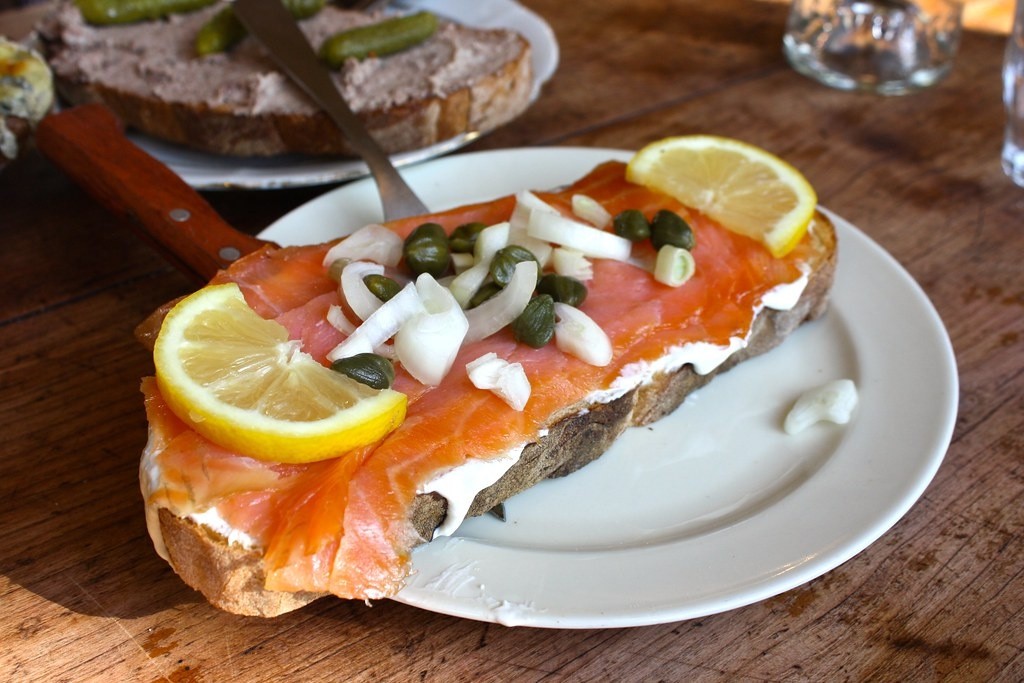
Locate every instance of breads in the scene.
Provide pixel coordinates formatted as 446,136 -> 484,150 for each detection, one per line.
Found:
30,0 -> 534,157
142,192 -> 840,616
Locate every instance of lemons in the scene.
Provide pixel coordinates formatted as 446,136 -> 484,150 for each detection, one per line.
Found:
154,280 -> 409,466
624,133 -> 817,258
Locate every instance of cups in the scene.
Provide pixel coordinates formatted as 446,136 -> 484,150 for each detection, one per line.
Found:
782,0 -> 966,96
1001,0 -> 1023,187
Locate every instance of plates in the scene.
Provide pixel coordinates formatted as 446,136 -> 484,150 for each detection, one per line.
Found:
253,147 -> 960,627
53,0 -> 561,195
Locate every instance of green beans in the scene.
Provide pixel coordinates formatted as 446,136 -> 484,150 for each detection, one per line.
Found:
77,0 -> 439,69
